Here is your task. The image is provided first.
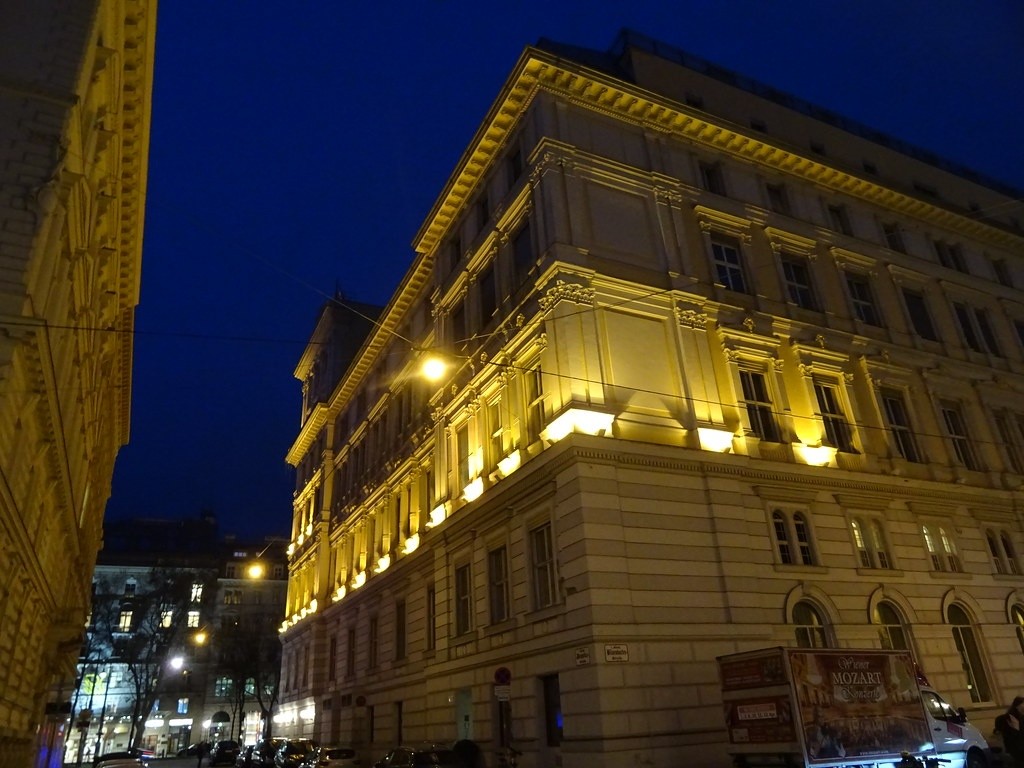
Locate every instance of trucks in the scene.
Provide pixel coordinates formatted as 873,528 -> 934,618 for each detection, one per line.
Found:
715,645 -> 993,768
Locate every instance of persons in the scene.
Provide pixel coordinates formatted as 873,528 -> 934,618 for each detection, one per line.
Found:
995,696 -> 1024,768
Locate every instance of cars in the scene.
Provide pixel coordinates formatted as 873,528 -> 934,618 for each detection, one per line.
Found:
208,740 -> 241,767
178,743 -> 213,758
298,744 -> 362,768
373,743 -> 467,768
235,737 -> 289,768
273,737 -> 315,768
